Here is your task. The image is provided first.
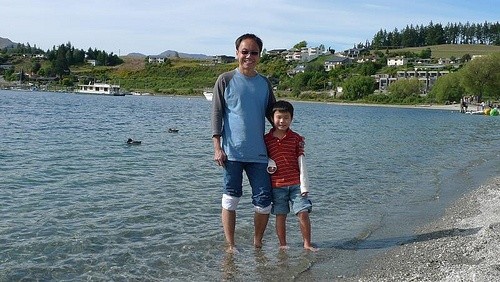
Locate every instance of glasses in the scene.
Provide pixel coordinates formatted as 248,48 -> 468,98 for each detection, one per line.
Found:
239,51 -> 260,55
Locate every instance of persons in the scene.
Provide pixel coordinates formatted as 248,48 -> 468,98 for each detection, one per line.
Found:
263,100 -> 320,252
210,34 -> 276,253
460,95 -> 500,114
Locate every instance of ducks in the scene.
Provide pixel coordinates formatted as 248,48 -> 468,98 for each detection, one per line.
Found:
127,138 -> 141,144
168,128 -> 179,133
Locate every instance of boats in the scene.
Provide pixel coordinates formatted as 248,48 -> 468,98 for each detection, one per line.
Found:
74,83 -> 125,96
203,92 -> 213,100
